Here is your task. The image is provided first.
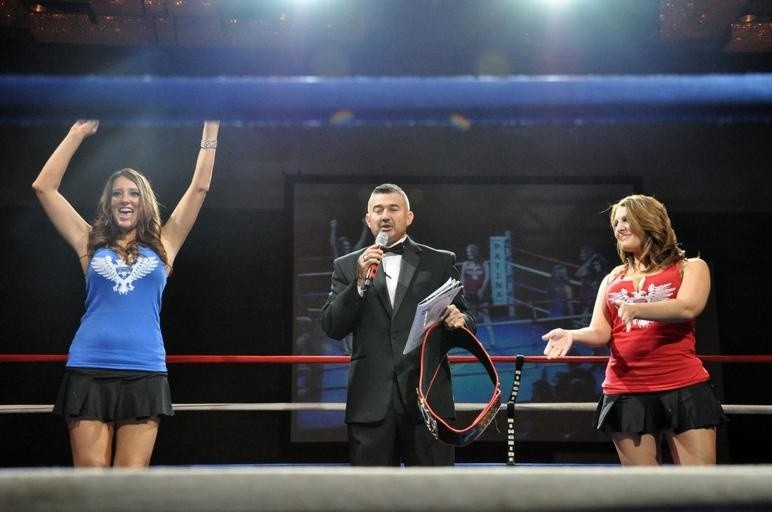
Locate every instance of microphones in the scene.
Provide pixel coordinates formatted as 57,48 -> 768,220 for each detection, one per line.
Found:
364,231 -> 388,292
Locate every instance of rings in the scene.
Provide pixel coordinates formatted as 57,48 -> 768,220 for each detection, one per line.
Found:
449,320 -> 456,324
363,254 -> 369,261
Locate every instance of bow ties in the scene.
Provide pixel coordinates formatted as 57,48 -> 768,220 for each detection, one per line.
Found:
383,243 -> 404,254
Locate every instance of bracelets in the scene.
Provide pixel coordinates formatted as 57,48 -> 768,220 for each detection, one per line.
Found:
199,139 -> 219,149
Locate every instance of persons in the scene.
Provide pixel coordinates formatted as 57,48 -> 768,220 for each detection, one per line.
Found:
327,214 -> 372,355
577,244 -> 595,314
320,181 -> 482,467
539,191 -> 730,467
574,253 -> 610,329
458,243 -> 501,348
546,261 -> 582,371
30,117 -> 222,469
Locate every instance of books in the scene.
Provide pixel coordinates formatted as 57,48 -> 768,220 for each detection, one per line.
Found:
402,277 -> 466,356
